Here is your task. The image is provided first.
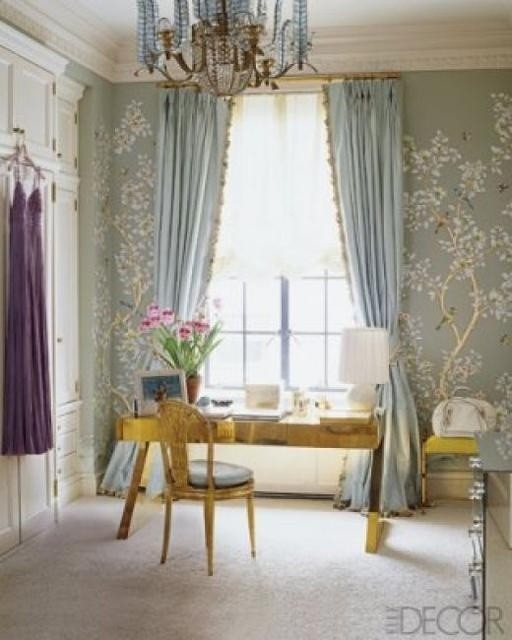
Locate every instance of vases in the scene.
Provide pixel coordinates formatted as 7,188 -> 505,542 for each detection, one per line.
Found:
186,377 -> 202,404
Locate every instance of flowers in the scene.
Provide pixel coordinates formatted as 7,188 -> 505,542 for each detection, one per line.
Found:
139,292 -> 225,378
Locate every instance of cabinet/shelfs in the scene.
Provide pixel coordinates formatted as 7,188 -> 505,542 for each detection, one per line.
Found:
470,431 -> 512,640
0,21 -> 70,567
56,76 -> 86,520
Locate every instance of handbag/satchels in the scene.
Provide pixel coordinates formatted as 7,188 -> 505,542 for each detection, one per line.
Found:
431,385 -> 497,439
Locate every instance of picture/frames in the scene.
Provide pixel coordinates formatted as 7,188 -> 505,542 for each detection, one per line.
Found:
136,368 -> 188,415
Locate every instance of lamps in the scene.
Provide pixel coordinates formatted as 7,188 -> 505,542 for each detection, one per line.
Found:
337,326 -> 389,413
130,0 -> 317,99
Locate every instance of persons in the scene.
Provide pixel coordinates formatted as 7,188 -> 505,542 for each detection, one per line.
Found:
155,383 -> 167,402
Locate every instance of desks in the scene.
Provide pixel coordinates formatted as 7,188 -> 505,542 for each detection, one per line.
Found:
115,408 -> 385,553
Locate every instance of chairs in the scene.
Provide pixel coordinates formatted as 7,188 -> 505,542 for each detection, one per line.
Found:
420,397 -> 496,506
153,400 -> 255,576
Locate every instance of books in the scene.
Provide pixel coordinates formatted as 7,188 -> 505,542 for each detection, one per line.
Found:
197,407 -> 282,421
319,412 -> 375,423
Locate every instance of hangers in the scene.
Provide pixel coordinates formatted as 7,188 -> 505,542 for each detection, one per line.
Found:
5,127 -> 44,180
